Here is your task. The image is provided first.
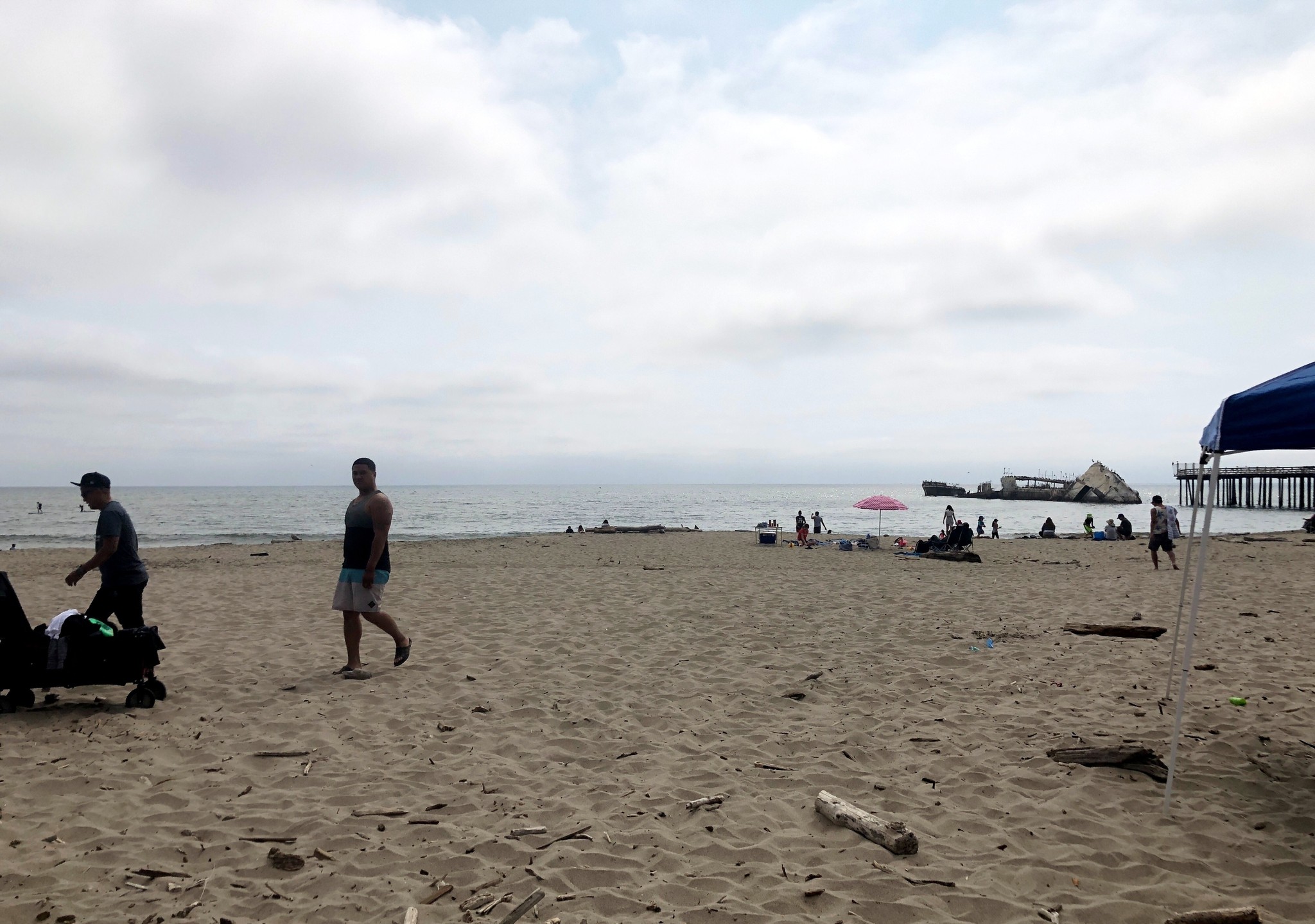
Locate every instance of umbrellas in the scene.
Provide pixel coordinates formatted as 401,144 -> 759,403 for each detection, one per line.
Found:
853,494 -> 909,541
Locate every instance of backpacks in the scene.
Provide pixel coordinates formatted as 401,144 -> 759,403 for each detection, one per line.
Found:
915,540 -> 924,554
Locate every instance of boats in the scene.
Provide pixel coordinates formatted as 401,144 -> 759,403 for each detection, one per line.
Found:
585,526 -> 615,534
1000,468 -> 1075,500
919,552 -> 982,563
922,479 -> 966,496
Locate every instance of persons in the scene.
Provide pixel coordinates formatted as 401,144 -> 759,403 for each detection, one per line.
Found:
578,525 -> 584,532
79,504 -> 83,512
566,526 -> 574,533
977,516 -> 986,537
10,544 -> 16,550
1105,519 -> 1117,541
65,472 -> 148,628
1084,514 -> 1095,538
37,502 -> 42,513
601,520 -> 610,528
939,530 -> 946,538
796,510 -> 806,532
943,505 -> 957,535
332,457 -> 412,675
992,519 -> 1001,539
811,511 -> 827,534
1148,496 -> 1179,570
1116,514 -> 1132,540
798,524 -> 816,547
1039,517 -> 1055,537
957,520 -> 963,526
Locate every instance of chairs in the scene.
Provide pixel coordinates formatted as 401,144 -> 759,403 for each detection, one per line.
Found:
927,526 -> 974,553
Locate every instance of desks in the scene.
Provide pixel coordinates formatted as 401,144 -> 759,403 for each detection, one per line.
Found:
755,527 -> 782,546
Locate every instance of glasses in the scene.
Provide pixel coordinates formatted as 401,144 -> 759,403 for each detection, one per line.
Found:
81,488 -> 97,499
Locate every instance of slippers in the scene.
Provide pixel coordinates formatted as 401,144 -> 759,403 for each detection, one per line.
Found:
899,545 -> 903,547
394,638 -> 412,666
805,546 -> 813,550
898,546 -> 903,549
333,666 -> 353,675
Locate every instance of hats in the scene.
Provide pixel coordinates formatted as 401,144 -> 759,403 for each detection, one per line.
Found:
70,472 -> 110,489
1117,514 -> 1124,519
978,516 -> 985,521
1151,495 -> 1162,503
1106,519 -> 1115,526
1087,514 -> 1093,518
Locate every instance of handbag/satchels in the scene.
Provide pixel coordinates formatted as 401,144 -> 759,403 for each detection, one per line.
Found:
839,540 -> 853,551
857,539 -> 869,549
114,626 -> 166,680
1094,531 -> 1104,538
865,535 -> 879,549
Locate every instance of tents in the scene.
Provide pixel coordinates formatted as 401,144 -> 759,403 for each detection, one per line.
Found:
1165,361 -> 1315,816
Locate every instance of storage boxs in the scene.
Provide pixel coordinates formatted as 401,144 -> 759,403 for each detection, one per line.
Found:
759,532 -> 776,544
1094,531 -> 1105,540
